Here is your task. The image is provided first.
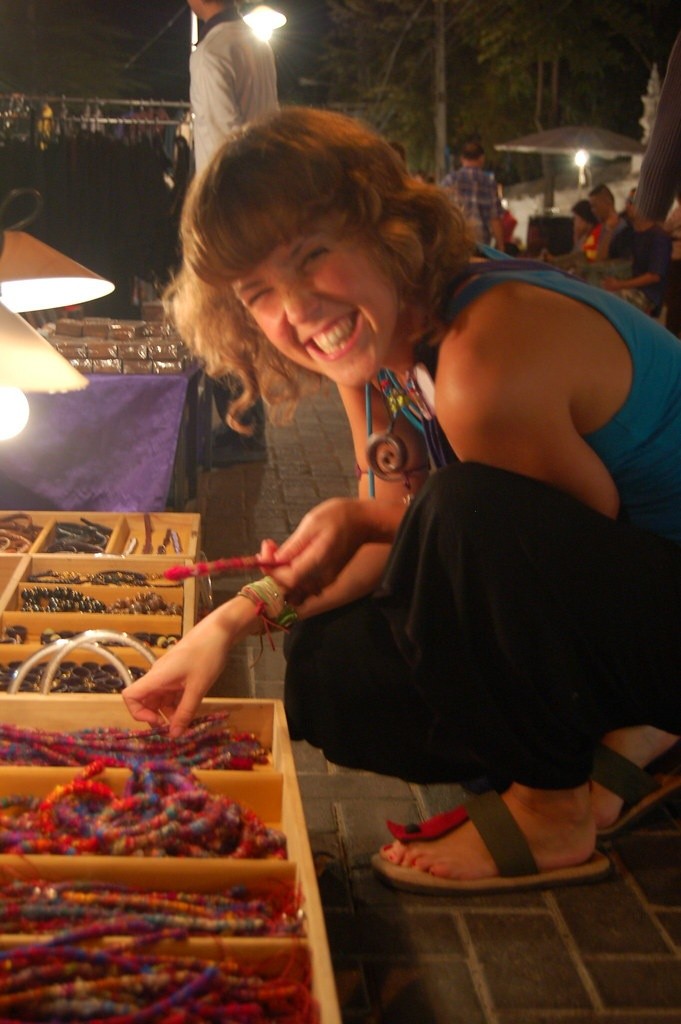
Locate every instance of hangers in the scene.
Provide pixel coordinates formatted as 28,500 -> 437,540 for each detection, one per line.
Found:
0,93 -> 185,150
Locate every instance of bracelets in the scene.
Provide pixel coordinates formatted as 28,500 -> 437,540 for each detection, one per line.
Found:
237,575 -> 298,670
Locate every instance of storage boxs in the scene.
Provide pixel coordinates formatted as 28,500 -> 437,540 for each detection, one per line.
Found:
0,697 -> 342,1024
0,554 -> 199,697
0,509 -> 202,563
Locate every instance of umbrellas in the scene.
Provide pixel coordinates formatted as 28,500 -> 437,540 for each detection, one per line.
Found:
495,125 -> 645,158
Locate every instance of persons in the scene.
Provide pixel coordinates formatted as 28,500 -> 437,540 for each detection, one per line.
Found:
184,0 -> 279,467
387,31 -> 681,339
120,105 -> 681,887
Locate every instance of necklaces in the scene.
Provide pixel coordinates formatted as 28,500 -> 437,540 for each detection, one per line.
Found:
377,311 -> 431,505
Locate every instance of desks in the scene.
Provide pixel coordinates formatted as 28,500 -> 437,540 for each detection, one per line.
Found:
0,361 -> 214,512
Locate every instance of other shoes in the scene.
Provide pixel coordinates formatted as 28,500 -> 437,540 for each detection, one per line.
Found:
212,425 -> 268,464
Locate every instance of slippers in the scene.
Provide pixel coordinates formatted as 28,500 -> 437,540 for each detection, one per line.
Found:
563,735 -> 681,842
367,787 -> 617,902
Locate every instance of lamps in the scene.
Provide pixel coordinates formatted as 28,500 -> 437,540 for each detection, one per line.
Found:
0,303 -> 90,440
0,231 -> 115,313
